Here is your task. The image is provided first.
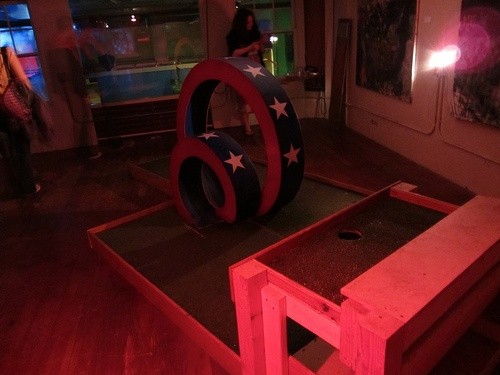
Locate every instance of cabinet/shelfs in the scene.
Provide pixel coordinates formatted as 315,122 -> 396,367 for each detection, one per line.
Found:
84,62 -> 199,141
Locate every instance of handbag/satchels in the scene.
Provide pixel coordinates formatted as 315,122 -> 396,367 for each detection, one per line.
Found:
27,95 -> 53,142
0,46 -> 32,122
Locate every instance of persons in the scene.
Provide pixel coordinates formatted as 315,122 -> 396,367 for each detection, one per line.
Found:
223,6 -> 269,138
0,44 -> 44,198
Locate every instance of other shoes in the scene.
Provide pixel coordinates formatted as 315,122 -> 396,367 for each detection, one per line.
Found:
246,130 -> 253,135
34,185 -> 40,193
